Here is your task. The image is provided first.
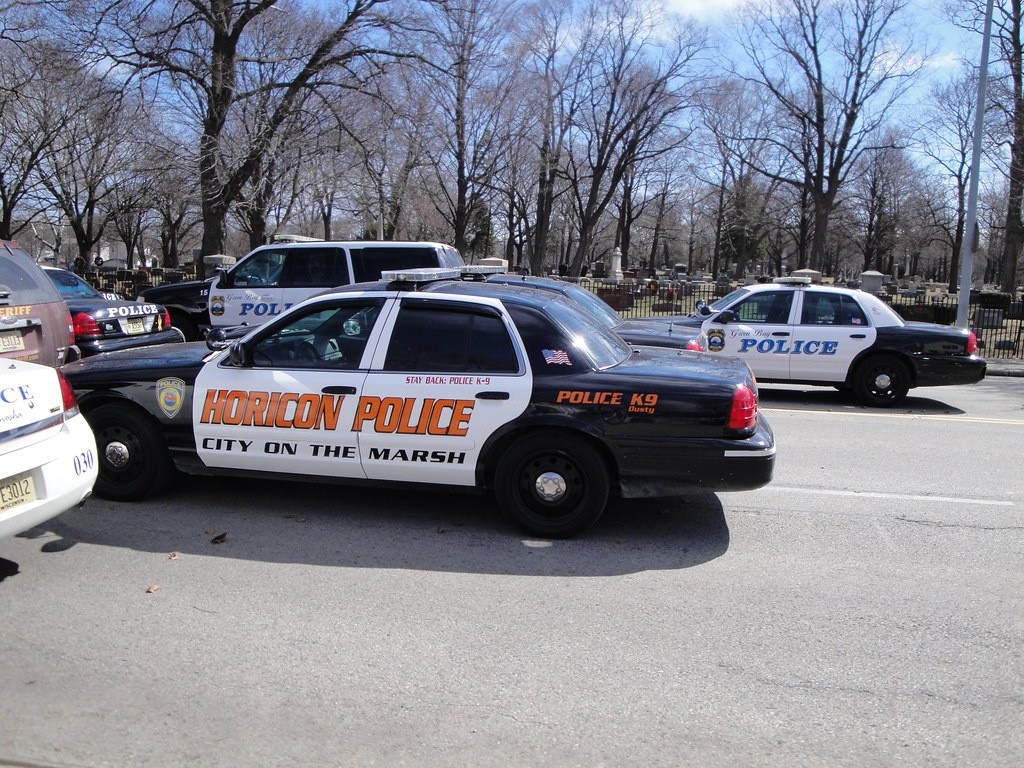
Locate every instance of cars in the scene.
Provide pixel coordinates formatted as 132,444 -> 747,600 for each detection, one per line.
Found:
626,277 -> 989,407
0,355 -> 98,549
454,266 -> 710,354
40,264 -> 187,365
59,269 -> 777,541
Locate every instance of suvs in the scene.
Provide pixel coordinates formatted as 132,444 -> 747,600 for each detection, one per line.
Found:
0,240 -> 80,369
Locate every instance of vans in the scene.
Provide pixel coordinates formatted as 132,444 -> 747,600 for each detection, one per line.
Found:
137,235 -> 464,332
